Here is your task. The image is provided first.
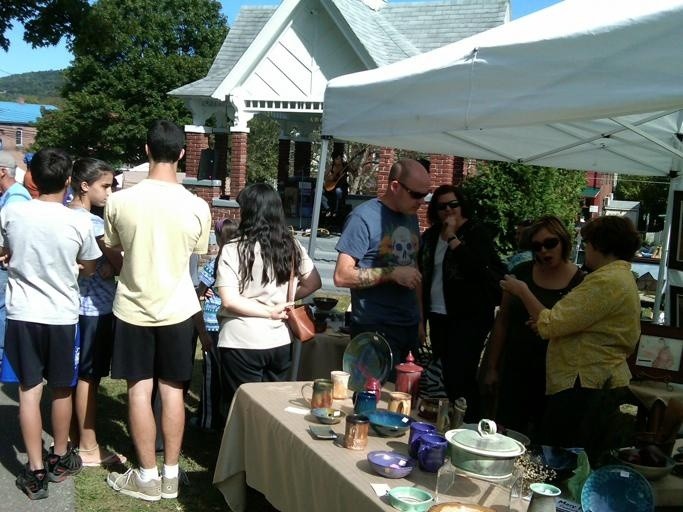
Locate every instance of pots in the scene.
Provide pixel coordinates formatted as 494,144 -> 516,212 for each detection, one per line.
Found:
444,418 -> 524,479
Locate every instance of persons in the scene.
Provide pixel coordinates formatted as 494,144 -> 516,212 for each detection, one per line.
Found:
193,218 -> 241,439
419,184 -> 503,420
480,213 -> 586,443
320,151 -> 356,217
0,149 -> 101,499
1,149 -> 126,467
330,157 -> 428,381
498,215 -> 643,466
101,120 -> 211,501
211,183 -> 321,457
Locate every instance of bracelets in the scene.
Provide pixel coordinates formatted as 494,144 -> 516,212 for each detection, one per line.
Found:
445,235 -> 456,243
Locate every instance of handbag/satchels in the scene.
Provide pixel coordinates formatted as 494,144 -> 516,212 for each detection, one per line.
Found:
286,304 -> 316,343
417,353 -> 449,399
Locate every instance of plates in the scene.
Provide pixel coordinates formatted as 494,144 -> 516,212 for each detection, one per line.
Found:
581,464 -> 655,511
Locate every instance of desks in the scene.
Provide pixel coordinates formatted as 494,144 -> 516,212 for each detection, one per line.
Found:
207,317 -> 682,511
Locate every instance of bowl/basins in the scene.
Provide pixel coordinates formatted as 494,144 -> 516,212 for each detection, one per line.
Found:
610,446 -> 675,479
462,421 -> 530,447
361,409 -> 416,436
312,297 -> 337,311
342,331 -> 393,391
312,408 -> 345,423
366,450 -> 416,478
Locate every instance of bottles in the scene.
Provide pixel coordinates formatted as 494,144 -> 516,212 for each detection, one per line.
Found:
452,394 -> 468,428
434,397 -> 450,434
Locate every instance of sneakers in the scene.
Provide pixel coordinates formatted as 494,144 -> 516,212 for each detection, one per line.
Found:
106,468 -> 161,501
46,445 -> 83,483
161,468 -> 191,499
16,462 -> 48,499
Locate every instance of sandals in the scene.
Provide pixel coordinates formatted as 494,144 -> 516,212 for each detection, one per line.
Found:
77,444 -> 127,467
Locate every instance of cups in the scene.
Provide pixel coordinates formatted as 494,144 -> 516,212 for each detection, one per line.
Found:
416,433 -> 447,473
351,390 -> 376,412
344,414 -> 369,450
329,370 -> 350,398
407,422 -> 436,456
301,378 -> 334,409
392,350 -> 424,409
528,481 -> 561,511
388,391 -> 412,417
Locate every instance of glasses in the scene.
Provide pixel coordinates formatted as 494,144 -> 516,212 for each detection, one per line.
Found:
398,182 -> 429,199
437,199 -> 459,210
531,237 -> 559,252
